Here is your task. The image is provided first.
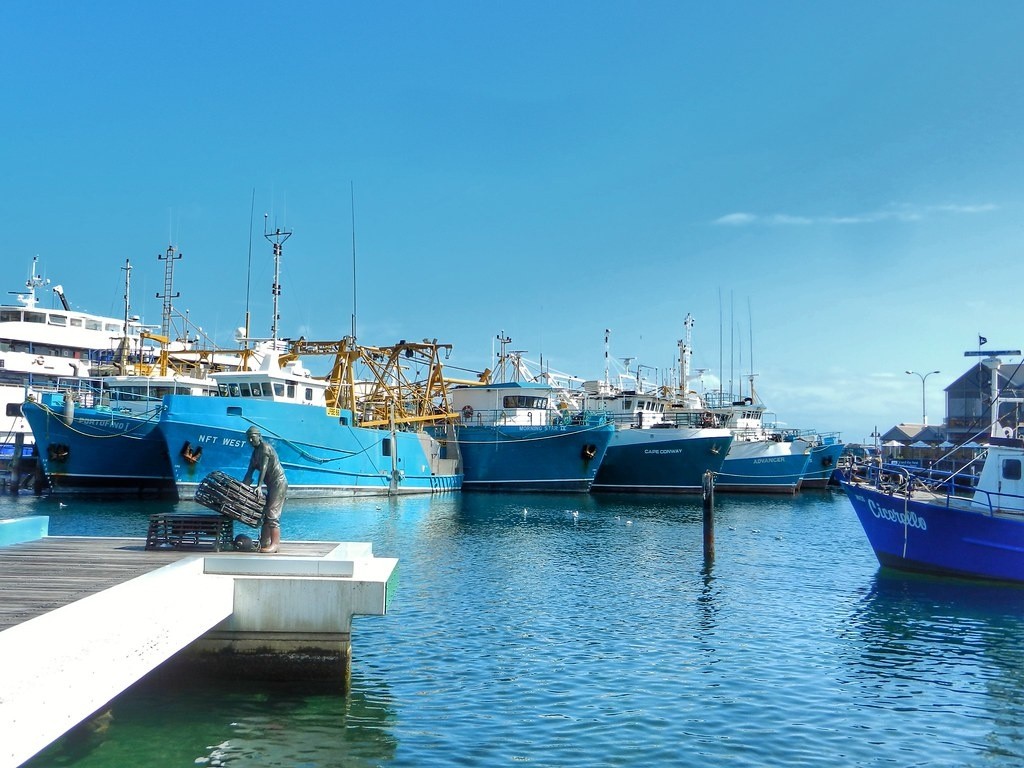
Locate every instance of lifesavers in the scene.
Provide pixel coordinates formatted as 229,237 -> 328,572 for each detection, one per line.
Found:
37,356 -> 45,365
762,431 -> 766,436
702,410 -> 715,423
64,351 -> 69,356
793,429 -> 798,437
462,405 -> 474,417
61,380 -> 67,385
850,455 -> 863,466
877,448 -> 881,455
583,442 -> 598,458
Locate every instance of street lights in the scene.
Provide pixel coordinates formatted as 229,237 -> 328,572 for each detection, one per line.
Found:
905,370 -> 942,427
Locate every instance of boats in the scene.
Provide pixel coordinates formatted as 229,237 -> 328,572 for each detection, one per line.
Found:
835,358 -> 1024,585
0,181 -> 878,502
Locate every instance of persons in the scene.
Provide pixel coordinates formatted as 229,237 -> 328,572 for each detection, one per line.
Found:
243,426 -> 288,553
